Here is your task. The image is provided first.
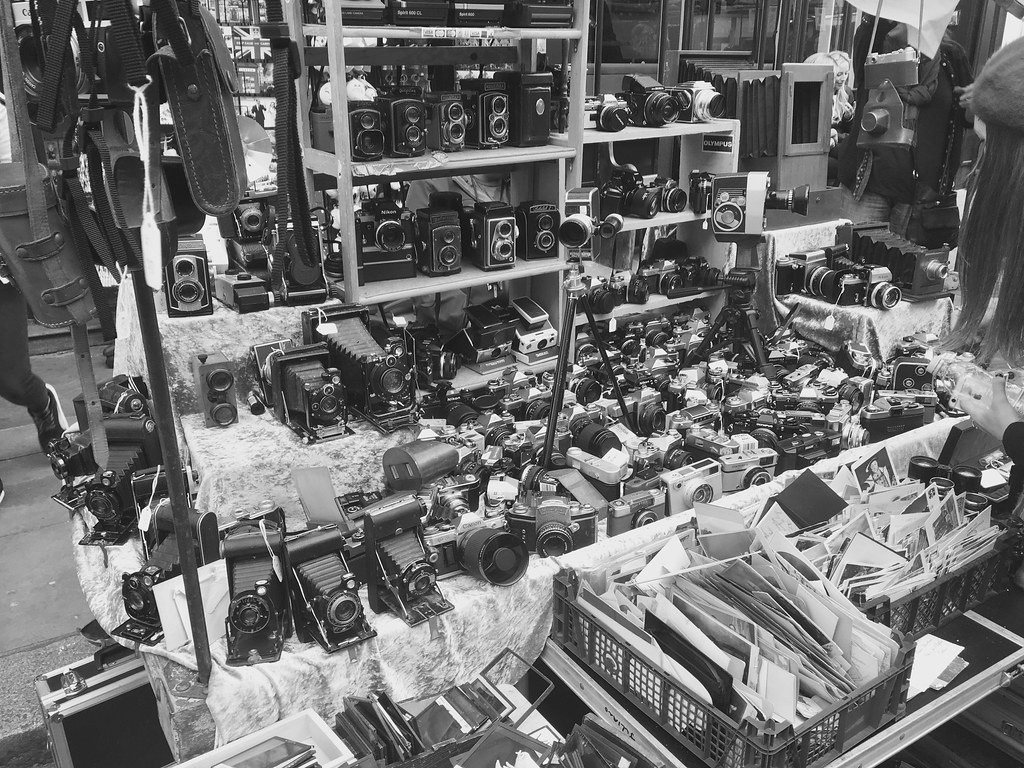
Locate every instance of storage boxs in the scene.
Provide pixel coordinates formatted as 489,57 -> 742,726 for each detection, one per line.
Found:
763,518 -> 1019,641
178,708 -> 354,768
551,517 -> 916,768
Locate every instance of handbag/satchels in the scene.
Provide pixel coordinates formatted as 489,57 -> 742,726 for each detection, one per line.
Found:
905,191 -> 961,251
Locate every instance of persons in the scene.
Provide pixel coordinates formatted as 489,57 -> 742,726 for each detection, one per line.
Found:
934,36 -> 1024,470
0,250 -> 69,503
802,0 -> 976,250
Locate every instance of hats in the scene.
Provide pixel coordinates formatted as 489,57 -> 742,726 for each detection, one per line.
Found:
969,36 -> 1024,129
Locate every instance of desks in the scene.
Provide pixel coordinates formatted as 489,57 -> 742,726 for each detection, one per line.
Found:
541,585 -> 1024,768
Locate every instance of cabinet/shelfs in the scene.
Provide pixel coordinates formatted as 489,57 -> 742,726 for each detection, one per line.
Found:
282,0 -> 738,394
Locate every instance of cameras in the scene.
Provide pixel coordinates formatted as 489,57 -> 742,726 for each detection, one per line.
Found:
53,63 -> 951,666
